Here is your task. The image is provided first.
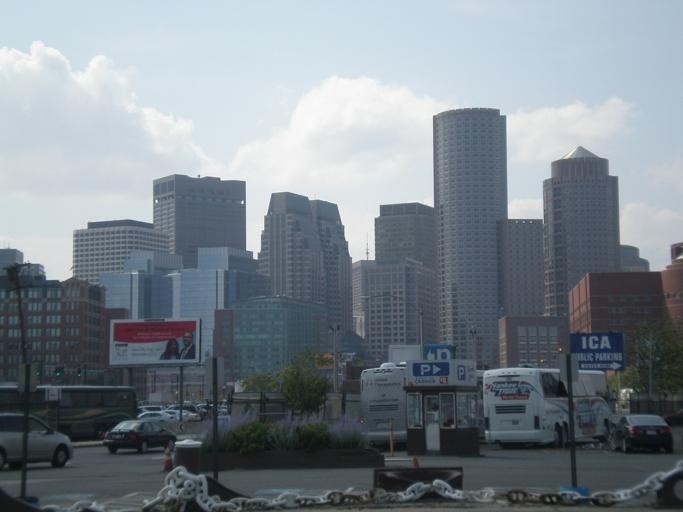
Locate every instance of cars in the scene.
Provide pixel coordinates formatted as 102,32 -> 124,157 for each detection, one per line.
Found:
136,399 -> 229,425
102,419 -> 176,452
607,414 -> 673,453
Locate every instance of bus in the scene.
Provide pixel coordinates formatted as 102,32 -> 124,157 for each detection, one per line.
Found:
0,384 -> 136,442
482,366 -> 618,447
358,360 -> 485,445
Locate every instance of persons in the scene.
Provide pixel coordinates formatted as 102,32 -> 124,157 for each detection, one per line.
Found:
180,332 -> 195,359
160,339 -> 180,360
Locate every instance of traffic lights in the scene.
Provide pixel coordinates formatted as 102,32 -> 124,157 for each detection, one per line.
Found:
35,369 -> 40,379
55,367 -> 60,376
76,367 -> 81,378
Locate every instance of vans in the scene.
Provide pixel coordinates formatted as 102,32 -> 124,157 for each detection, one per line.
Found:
0,412 -> 73,468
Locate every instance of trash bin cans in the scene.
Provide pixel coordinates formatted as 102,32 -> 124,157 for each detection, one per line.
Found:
175,439 -> 203,476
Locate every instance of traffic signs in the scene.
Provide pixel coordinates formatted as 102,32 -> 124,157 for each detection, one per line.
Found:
568,331 -> 624,370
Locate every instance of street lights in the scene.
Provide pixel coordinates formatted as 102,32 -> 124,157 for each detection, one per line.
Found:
360,294 -> 423,359
328,323 -> 341,392
470,328 -> 478,358
